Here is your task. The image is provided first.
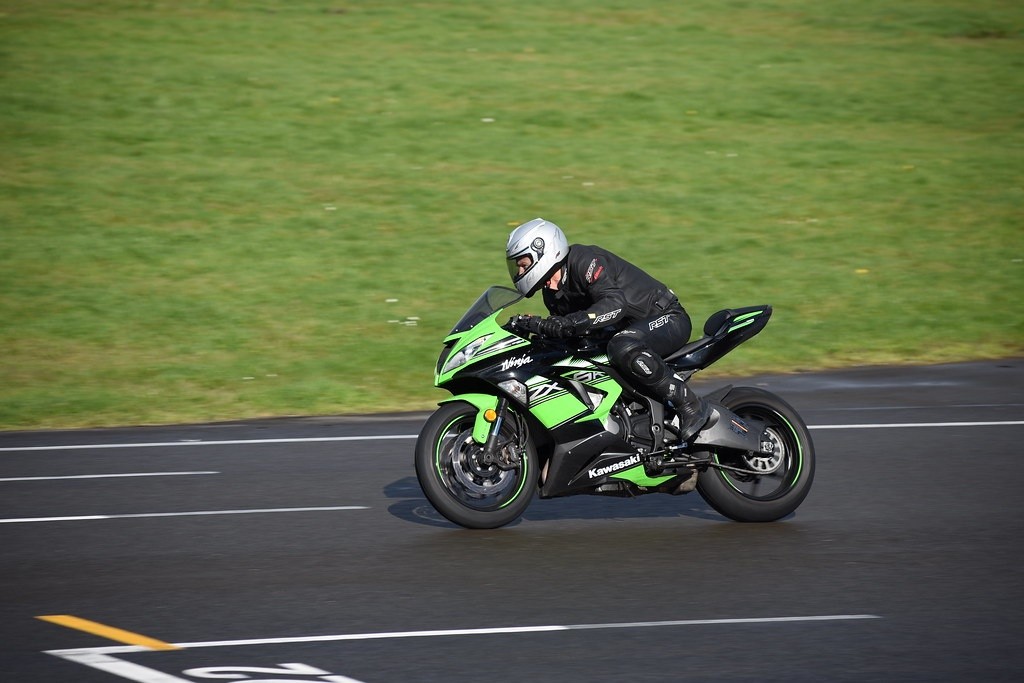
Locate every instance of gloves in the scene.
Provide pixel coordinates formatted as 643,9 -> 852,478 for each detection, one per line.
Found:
541,310 -> 590,342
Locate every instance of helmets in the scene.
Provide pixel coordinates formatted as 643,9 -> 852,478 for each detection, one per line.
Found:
505,217 -> 569,298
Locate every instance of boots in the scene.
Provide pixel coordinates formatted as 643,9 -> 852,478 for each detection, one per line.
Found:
651,368 -> 712,441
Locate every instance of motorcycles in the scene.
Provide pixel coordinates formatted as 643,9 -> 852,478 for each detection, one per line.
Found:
412,286 -> 816,531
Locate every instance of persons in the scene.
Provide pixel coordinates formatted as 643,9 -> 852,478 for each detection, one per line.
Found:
507,219 -> 714,441
